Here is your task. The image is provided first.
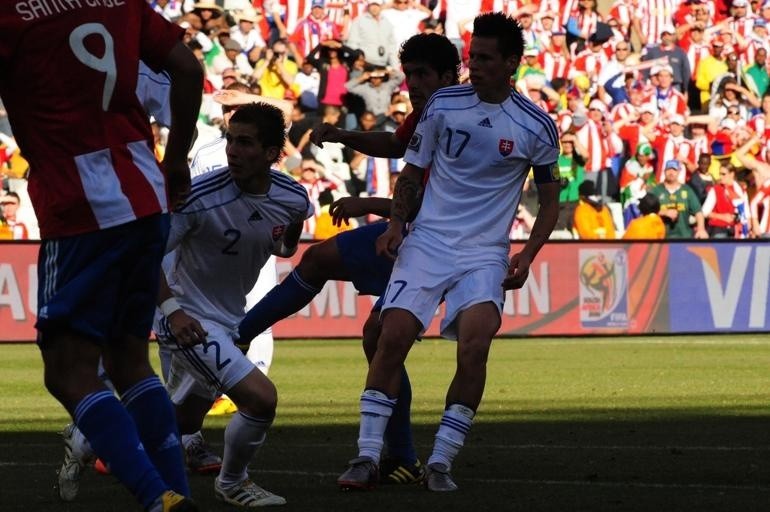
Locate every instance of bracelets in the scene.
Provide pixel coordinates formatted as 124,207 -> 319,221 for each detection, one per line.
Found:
279,243 -> 298,256
161,295 -> 183,317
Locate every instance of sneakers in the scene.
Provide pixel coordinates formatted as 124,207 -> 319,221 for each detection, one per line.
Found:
336,456 -> 459,493
53,421 -> 288,512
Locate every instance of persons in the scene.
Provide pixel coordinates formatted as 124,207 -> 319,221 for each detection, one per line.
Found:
0,2 -> 769,241
2,2 -> 202,512
60,102 -> 312,506
333,9 -> 560,496
191,83 -> 301,415
231,34 -> 461,486
90,245 -> 221,471
